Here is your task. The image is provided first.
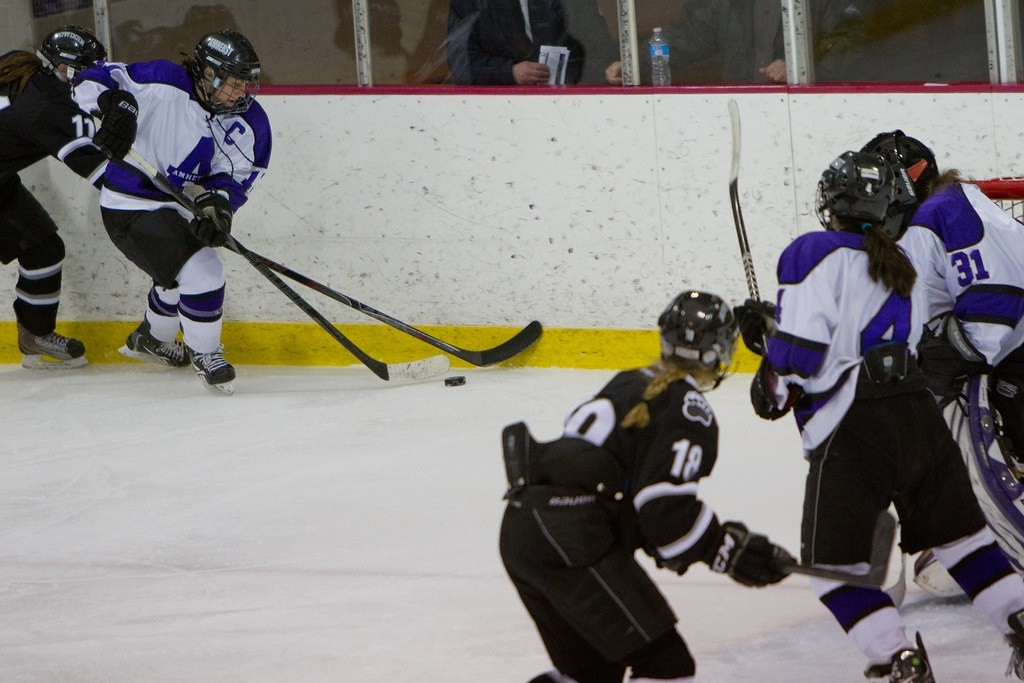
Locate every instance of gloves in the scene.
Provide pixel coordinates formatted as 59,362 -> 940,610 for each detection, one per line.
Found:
750,357 -> 793,419
191,193 -> 232,247
712,522 -> 800,587
733,300 -> 780,356
92,89 -> 139,162
915,337 -> 968,398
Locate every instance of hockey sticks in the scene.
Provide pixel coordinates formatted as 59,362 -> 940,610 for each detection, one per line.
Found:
130,148 -> 451,384
221,243 -> 544,369
780,511 -> 898,588
726,96 -> 773,355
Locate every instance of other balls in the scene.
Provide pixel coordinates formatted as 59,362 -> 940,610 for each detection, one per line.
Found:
445,376 -> 467,387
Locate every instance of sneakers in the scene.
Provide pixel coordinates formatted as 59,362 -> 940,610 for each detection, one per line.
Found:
1005,609 -> 1024,678
18,332 -> 87,369
866,650 -> 936,683
188,350 -> 238,395
119,331 -> 191,368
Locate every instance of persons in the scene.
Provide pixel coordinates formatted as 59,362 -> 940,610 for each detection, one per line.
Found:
63,30 -> 272,386
604,0 -> 871,87
0,25 -> 109,360
733,129 -> 1024,683
498,291 -> 798,683
446,0 -> 620,86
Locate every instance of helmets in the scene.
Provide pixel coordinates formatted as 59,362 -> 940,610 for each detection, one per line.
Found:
859,129 -> 938,206
36,25 -> 107,83
816,151 -> 896,231
190,30 -> 261,114
658,291 -> 739,392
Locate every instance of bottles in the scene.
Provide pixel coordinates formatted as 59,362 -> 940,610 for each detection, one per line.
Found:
648,27 -> 672,86
